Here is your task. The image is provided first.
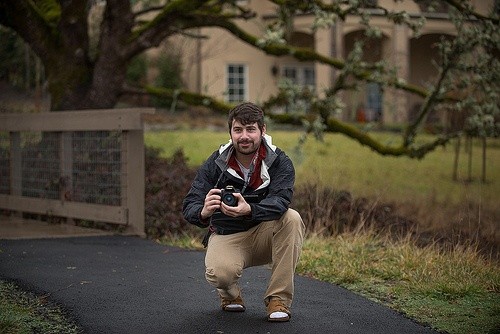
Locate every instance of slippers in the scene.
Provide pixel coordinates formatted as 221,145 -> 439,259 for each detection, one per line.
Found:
267,298 -> 292,321
220,290 -> 246,312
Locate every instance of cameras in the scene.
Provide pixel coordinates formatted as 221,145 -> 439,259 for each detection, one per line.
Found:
213,185 -> 240,206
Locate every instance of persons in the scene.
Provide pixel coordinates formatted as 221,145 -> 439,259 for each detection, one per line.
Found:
181,102 -> 306,322
